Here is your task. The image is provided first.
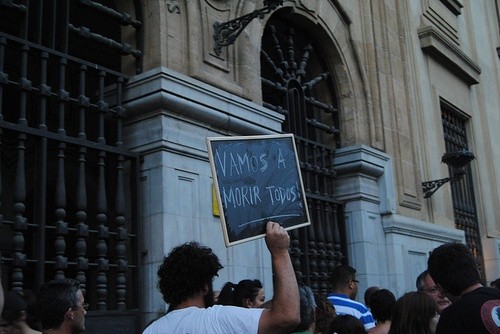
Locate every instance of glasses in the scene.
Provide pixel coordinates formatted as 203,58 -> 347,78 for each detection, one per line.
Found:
74,303 -> 89,311
346,279 -> 360,284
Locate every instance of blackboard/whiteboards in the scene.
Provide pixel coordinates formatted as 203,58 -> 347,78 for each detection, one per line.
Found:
204,133 -> 312,249
